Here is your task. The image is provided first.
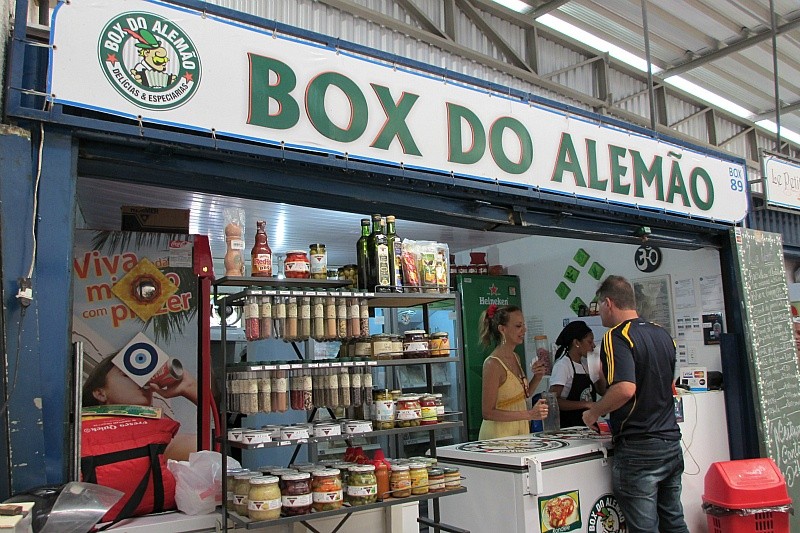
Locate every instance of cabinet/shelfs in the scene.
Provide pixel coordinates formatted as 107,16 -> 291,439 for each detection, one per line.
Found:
211,273 -> 472,533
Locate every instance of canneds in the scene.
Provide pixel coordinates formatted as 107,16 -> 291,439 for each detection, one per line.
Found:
284,243 -> 358,292
227,330 -> 461,522
145,357 -> 183,388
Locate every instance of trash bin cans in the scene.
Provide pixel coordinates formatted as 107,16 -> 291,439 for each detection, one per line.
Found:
703,457 -> 794,533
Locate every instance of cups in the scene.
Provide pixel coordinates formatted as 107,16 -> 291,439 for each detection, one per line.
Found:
541,392 -> 561,434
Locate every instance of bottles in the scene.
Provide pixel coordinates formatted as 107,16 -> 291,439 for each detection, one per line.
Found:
226,213 -> 462,519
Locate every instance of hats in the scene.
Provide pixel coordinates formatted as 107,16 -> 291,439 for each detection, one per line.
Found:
554,319 -> 592,348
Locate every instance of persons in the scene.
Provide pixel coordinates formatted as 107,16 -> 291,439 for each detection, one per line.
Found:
82,350 -> 198,461
550,321 -> 608,429
582,275 -> 690,533
478,304 -> 549,442
